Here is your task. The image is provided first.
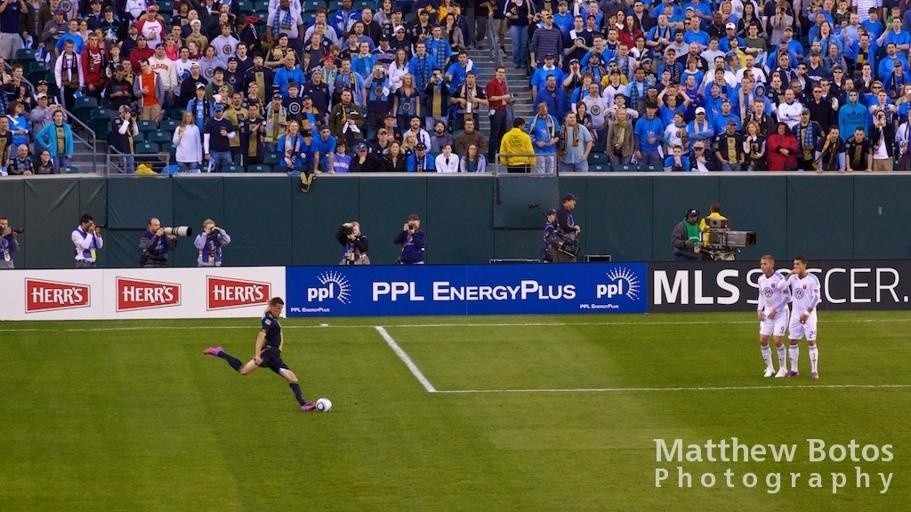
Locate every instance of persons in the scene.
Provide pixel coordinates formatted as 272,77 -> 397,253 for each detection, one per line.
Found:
757,254 -> 796,379
193,217 -> 233,269
394,213 -> 429,263
70,214 -> 104,269
1,2 -> 909,173
334,221 -> 371,264
671,208 -> 704,260
137,217 -> 177,267
778,255 -> 821,378
700,201 -> 728,247
1,217 -> 22,269
556,192 -> 583,264
539,208 -> 557,262
202,295 -> 316,411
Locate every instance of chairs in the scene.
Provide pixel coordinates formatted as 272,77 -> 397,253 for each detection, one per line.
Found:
0,0 -> 911,174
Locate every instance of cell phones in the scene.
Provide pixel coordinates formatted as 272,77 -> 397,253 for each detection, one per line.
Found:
432,73 -> 436,78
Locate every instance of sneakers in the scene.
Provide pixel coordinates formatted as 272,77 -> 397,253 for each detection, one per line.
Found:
300,404 -> 315,411
203,347 -> 224,356
764,368 -> 799,378
811,372 -> 819,379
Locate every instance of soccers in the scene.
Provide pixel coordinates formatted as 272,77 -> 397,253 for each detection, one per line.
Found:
315,397 -> 332,414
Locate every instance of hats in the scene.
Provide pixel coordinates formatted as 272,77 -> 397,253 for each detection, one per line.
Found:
647,102 -> 659,108
379,129 -> 387,134
156,44 -> 165,49
390,7 -> 401,15
546,209 -> 557,215
189,61 -> 199,69
569,58 -> 579,64
728,120 -> 736,125
147,6 -> 156,12
724,51 -> 736,60
229,57 -> 238,63
726,22 -> 736,30
561,193 -> 580,200
416,142 -> 427,149
380,35 -> 388,41
642,51 -> 653,64
382,112 -> 395,120
356,143 -> 368,154
190,20 -> 201,26
696,107 -> 705,115
213,104 -> 224,112
542,8 -> 552,18
687,208 -> 697,216
785,26 -> 793,31
395,25 -> 405,33
608,57 -> 619,65
694,142 -> 704,148
545,53 -> 555,59
36,93 -> 48,102
195,82 -> 205,89
119,105 -> 130,112
37,80 -> 49,86
55,8 -> 64,15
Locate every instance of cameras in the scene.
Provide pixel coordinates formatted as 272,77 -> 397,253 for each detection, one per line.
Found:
93,223 -> 107,228
345,227 -> 353,235
210,227 -> 219,235
408,223 -> 414,229
130,111 -> 136,116
7,225 -> 23,236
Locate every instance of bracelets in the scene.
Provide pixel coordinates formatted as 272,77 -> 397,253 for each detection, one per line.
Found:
804,310 -> 811,316
